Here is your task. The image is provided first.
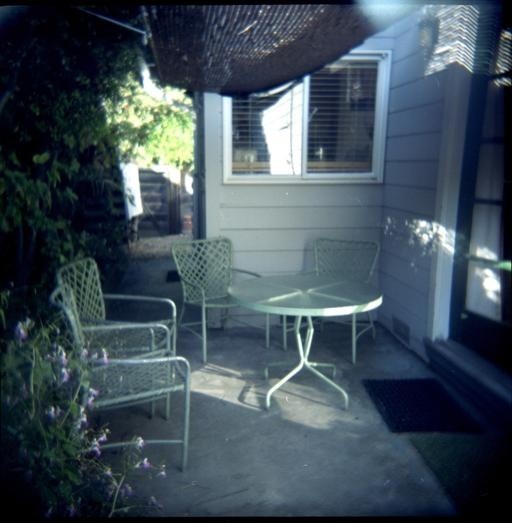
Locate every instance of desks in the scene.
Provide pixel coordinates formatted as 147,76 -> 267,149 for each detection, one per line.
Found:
228,275 -> 385,408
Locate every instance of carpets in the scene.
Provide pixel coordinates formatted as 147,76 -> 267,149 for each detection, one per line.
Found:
359,376 -> 483,433
411,433 -> 512,495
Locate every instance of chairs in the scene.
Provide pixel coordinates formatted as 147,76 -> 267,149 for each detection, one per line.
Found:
53,256 -> 178,410
50,284 -> 172,426
46,284 -> 191,473
282,235 -> 383,365
173,237 -> 270,364
